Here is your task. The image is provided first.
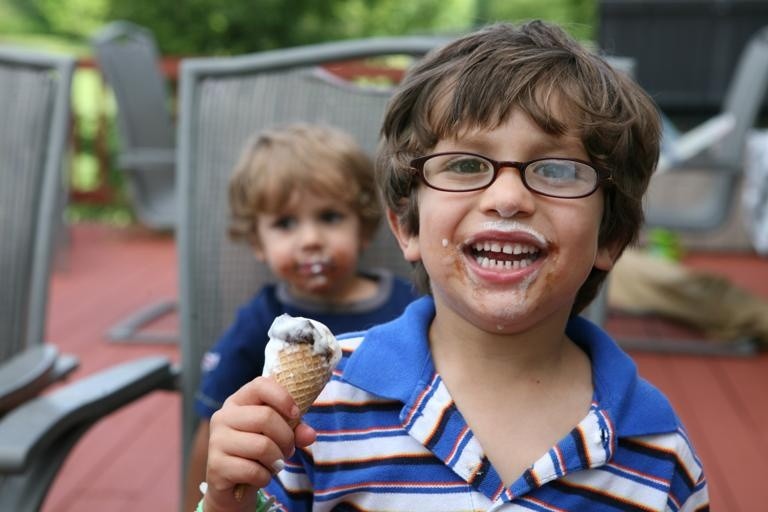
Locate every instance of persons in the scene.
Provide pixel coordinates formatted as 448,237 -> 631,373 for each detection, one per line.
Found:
193,17 -> 709,512
173,117 -> 424,512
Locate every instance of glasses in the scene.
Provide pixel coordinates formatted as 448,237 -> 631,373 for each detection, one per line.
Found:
409,151 -> 610,199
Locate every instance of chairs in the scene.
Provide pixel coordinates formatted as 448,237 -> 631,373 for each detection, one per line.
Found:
0,21 -> 453,512
577,29 -> 768,359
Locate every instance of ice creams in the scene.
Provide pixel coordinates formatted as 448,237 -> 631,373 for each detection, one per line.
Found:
234,313 -> 343,502
